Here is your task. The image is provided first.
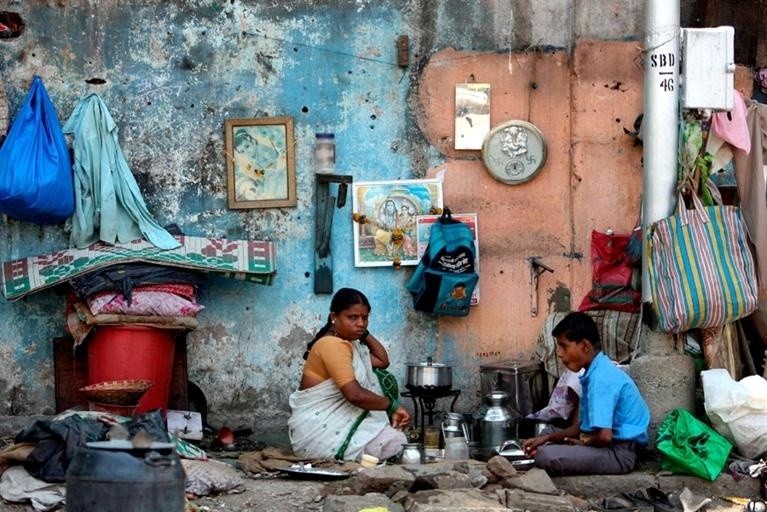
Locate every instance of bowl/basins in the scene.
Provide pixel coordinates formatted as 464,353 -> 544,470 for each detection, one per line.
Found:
510,459 -> 534,470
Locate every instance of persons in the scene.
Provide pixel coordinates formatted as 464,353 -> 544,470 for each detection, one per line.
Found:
394,204 -> 418,257
372,196 -> 401,257
286,285 -> 411,463
234,131 -> 279,175
521,311 -> 651,477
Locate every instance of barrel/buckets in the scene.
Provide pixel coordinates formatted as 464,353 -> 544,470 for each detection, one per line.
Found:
88,325 -> 176,419
65,440 -> 185,512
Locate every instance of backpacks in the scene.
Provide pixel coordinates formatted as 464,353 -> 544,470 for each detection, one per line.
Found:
405,208 -> 479,317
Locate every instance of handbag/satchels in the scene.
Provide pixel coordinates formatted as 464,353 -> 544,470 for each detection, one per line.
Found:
648,186 -> 759,333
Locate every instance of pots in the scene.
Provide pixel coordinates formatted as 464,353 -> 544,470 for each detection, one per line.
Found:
405,357 -> 453,392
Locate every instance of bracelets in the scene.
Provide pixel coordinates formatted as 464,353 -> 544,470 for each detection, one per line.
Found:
386,398 -> 398,414
359,328 -> 369,343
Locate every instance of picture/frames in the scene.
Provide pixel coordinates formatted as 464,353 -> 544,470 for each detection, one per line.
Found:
224,116 -> 296,210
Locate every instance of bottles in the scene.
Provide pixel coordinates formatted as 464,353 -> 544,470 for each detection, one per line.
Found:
399,438 -> 469,471
313,132 -> 336,176
